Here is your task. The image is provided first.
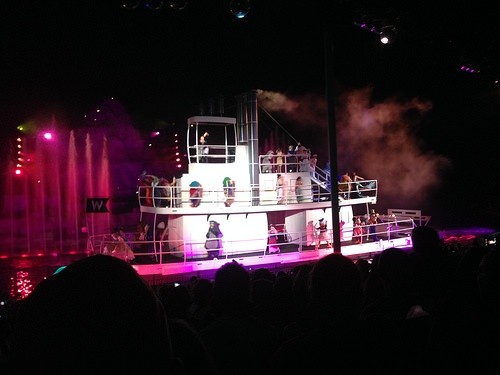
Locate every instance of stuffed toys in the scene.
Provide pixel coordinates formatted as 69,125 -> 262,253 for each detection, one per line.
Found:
204,220 -> 224,260
199,132 -> 210,163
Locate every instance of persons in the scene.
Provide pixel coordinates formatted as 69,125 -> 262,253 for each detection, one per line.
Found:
0,225 -> 500,375
261,143 -> 365,204
267,209 -> 383,254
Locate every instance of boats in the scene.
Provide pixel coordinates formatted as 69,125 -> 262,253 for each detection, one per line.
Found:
50,92 -> 425,283
